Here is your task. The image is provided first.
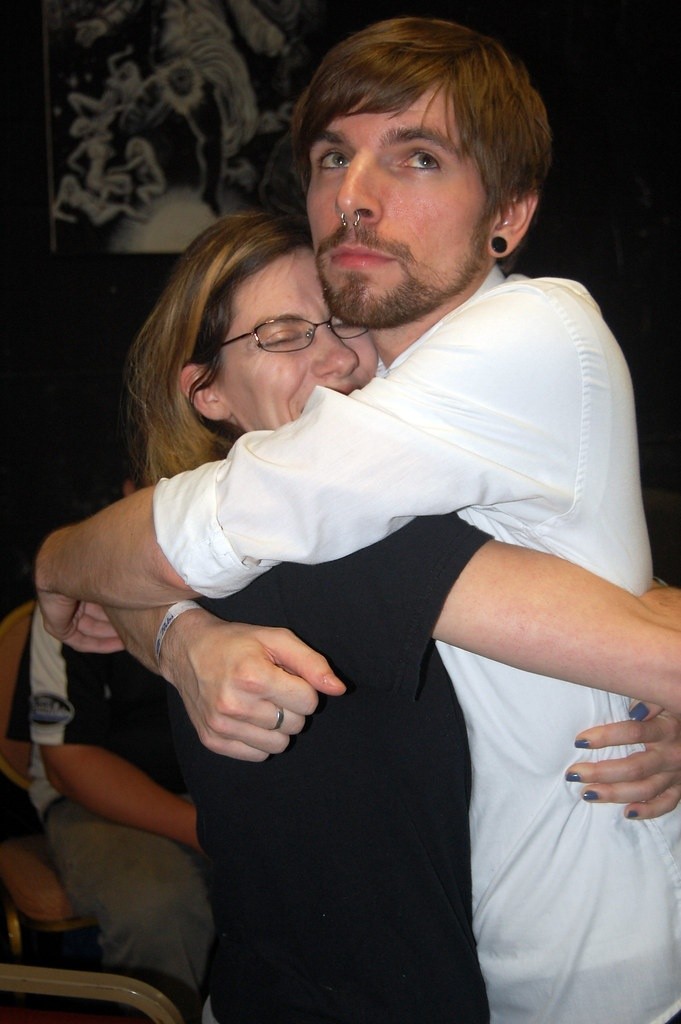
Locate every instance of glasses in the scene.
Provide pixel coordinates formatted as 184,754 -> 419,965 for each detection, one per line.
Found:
197,313 -> 370,365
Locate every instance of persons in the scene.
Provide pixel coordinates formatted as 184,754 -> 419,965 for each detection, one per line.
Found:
34,17 -> 681,1024
122,214 -> 681,1024
6,443 -> 213,1024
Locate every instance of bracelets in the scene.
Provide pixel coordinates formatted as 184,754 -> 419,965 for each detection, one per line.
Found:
154,599 -> 202,668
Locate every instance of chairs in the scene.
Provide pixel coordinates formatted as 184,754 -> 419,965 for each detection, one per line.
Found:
0,599 -> 99,956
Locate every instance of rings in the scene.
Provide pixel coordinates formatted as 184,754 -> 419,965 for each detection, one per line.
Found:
270,707 -> 285,731
